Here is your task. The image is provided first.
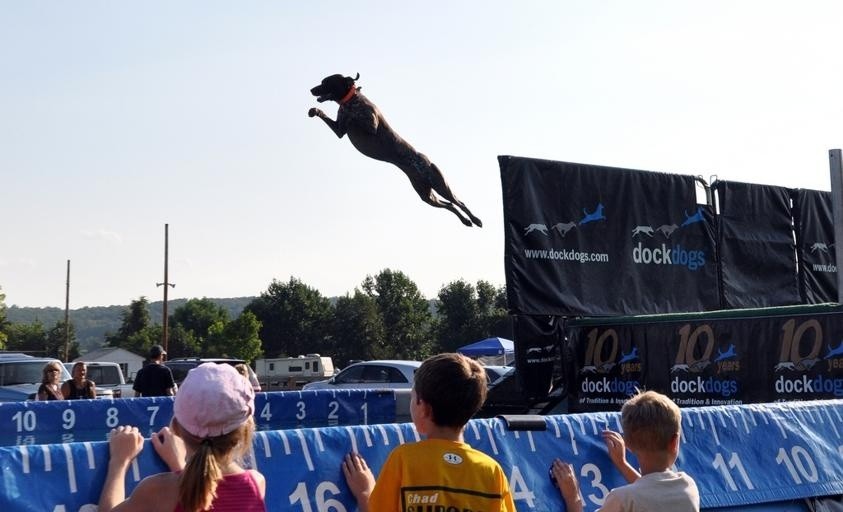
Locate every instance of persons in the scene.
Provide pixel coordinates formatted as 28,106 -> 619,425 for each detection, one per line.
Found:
99,363 -> 267,512
552,390 -> 700,511
62,361 -> 96,399
132,344 -> 178,396
37,360 -> 64,400
342,353 -> 519,512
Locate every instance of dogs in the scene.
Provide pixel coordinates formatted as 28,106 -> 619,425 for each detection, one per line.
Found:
306,72 -> 485,230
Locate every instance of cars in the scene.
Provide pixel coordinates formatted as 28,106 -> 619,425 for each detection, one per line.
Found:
164,357 -> 262,392
302,359 -> 514,391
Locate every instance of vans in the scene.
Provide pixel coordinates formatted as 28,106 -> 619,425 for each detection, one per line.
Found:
0,352 -> 143,401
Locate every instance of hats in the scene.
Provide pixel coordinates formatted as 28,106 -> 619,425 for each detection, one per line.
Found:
172,360 -> 254,440
150,344 -> 167,355
